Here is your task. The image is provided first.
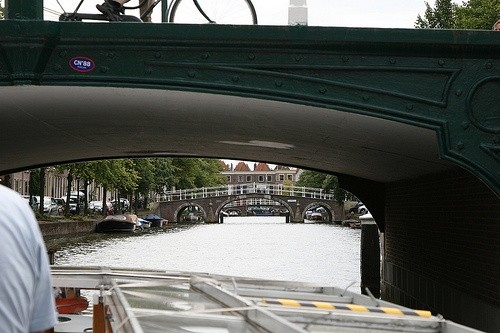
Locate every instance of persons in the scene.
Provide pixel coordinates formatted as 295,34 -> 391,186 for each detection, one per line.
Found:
0,183 -> 57,333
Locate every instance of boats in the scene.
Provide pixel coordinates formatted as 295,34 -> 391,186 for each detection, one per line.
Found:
95,215 -> 169,231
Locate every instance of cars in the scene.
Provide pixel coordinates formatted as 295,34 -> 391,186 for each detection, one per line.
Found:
29,192 -> 131,215
